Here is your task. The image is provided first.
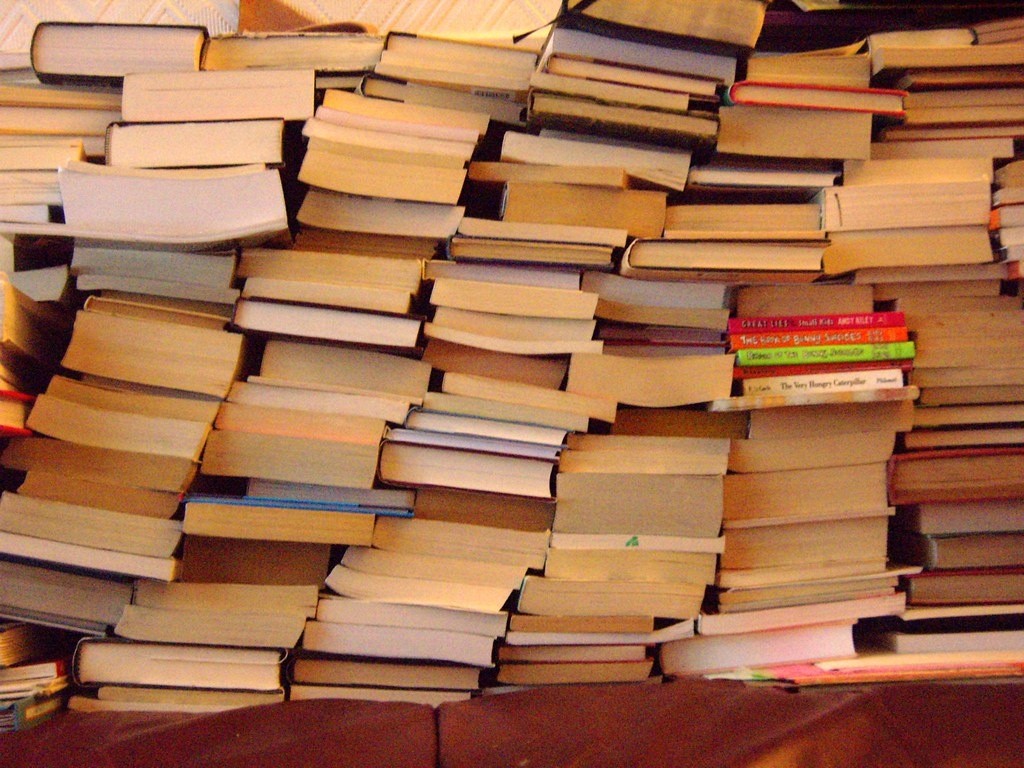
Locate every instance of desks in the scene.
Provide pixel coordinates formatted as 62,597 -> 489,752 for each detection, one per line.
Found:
0,667 -> 1024,768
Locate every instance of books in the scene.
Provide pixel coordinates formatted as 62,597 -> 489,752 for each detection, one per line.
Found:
0,0 -> 1024,732
